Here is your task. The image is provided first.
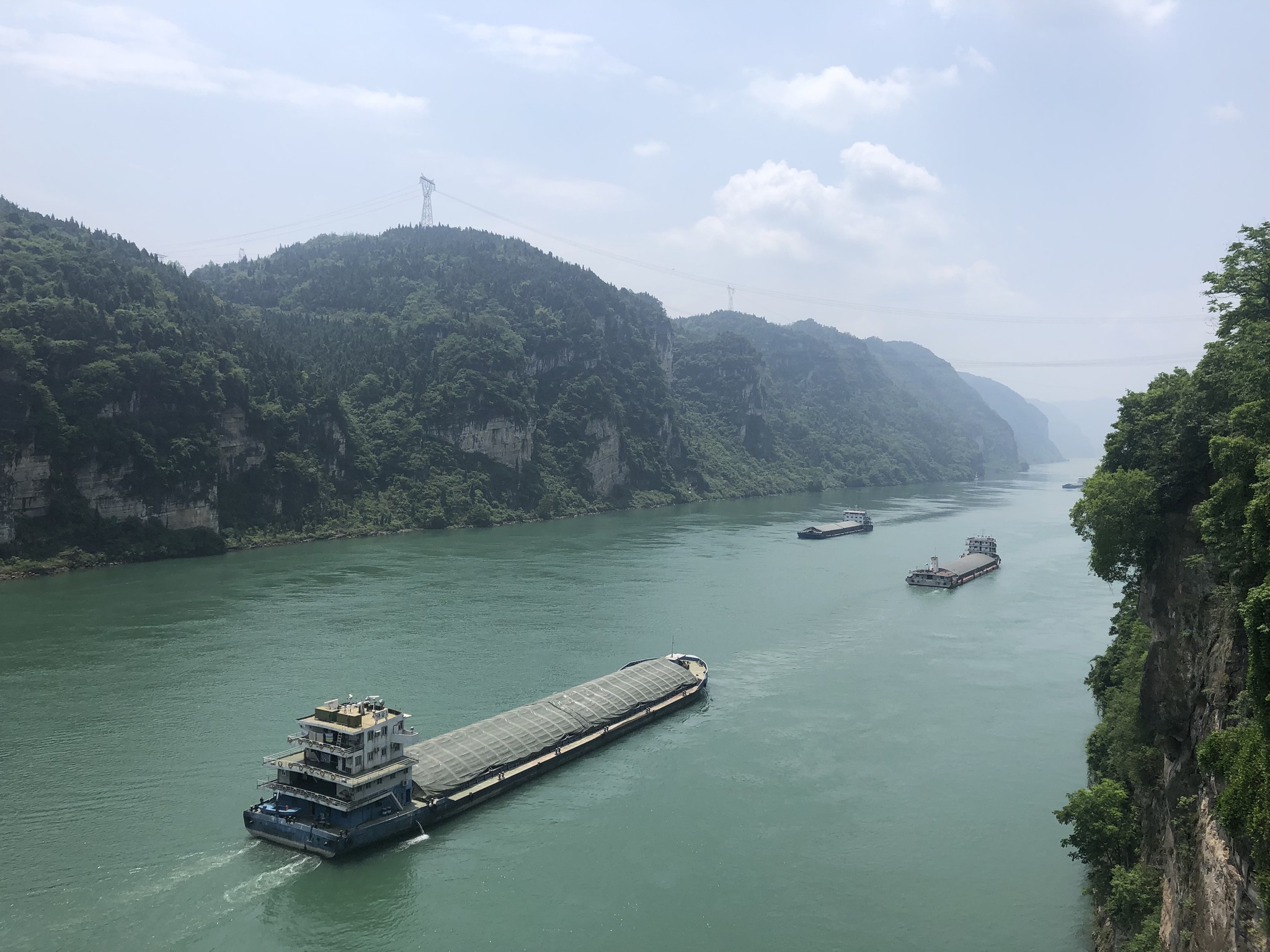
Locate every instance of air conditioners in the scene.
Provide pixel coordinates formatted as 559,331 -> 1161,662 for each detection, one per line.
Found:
375,747 -> 380,752
402,779 -> 409,786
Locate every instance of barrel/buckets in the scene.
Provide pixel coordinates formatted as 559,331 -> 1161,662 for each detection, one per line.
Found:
386,806 -> 391,814
382,808 -> 387,816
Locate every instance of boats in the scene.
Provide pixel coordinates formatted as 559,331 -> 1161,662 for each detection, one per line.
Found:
1062,475 -> 1088,488
975,472 -> 979,480
905,529 -> 1001,589
243,635 -> 708,858
797,504 -> 873,539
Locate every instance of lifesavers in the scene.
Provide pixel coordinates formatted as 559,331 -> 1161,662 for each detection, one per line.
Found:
292,788 -> 298,794
287,737 -> 292,743
288,763 -> 293,769
309,767 -> 314,774
272,784 -> 276,790
340,749 -> 345,755
331,773 -> 336,780
308,741 -> 313,747
282,786 -> 287,792
329,746 -> 334,752
297,738 -> 303,745
319,743 -> 324,750
303,791 -> 308,797
325,797 -> 330,804
298,764 -> 303,771
320,769 -> 325,777
314,794 -> 319,800
278,761 -> 282,767
